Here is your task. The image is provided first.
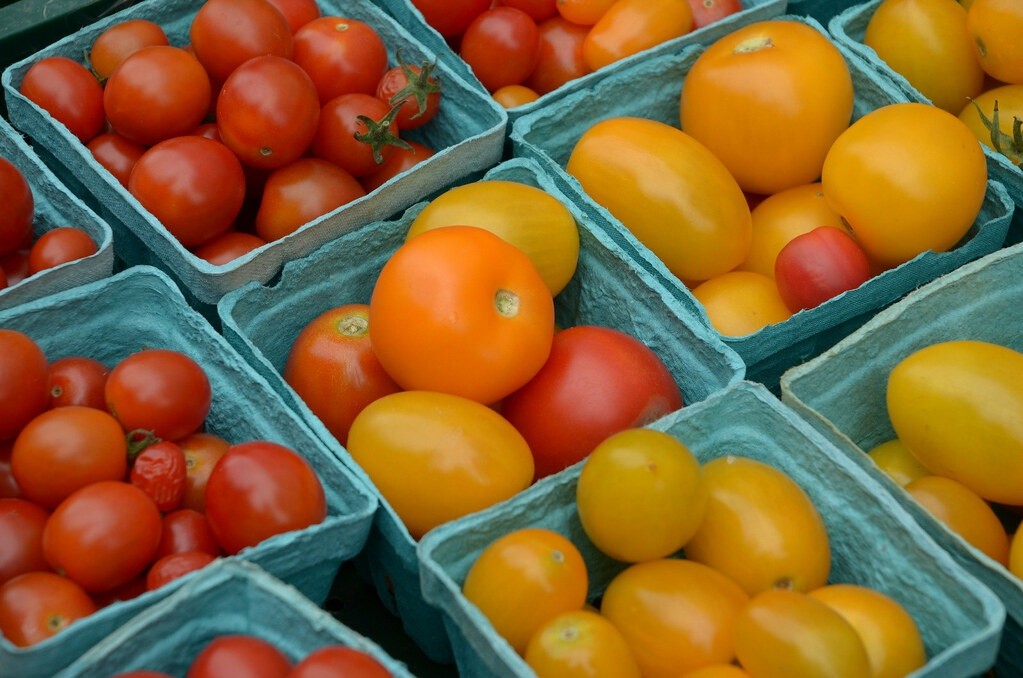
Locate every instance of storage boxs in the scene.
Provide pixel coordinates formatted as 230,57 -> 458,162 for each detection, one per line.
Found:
0,2 -> 1020,678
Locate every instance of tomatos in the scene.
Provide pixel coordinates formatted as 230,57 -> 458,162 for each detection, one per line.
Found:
0,0 -> 1022,678
